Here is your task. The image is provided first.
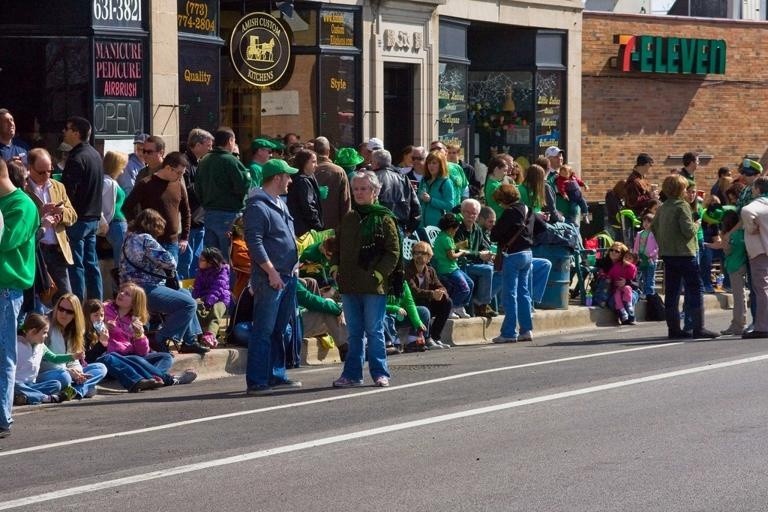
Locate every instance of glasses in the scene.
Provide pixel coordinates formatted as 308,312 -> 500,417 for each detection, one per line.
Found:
142,148 -> 158,156
170,169 -> 185,176
65,126 -> 72,132
608,248 -> 620,253
413,250 -> 430,256
232,152 -> 241,157
57,305 -> 75,315
411,156 -> 424,161
430,147 -> 441,152
31,166 -> 55,175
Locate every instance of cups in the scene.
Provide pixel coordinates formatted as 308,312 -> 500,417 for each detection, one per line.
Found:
650,184 -> 658,191
598,237 -> 604,248
409,180 -> 418,192
587,255 -> 596,266
318,186 -> 328,200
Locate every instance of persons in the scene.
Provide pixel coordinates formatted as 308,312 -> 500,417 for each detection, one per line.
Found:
0,109 -> 768,440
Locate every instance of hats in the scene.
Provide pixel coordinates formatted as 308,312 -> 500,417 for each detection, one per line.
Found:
704,203 -> 724,225
132,131 -> 150,145
262,158 -> 300,179
334,147 -> 365,167
251,135 -> 288,150
545,146 -> 565,158
616,210 -> 642,225
737,160 -> 764,174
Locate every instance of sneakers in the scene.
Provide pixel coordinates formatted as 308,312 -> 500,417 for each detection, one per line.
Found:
516,330 -> 532,342
704,282 -> 732,293
181,340 -> 210,354
669,323 -> 768,339
41,386 -> 77,404
333,377 -> 364,388
14,395 -> 26,404
375,375 -> 389,387
246,383 -> 274,395
618,306 -> 637,325
270,379 -> 302,389
151,341 -> 175,359
130,374 -> 174,393
492,335 -> 518,343
385,337 -> 450,354
448,303 -> 498,319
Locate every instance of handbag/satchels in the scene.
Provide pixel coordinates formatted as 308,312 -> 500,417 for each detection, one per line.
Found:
545,221 -> 576,251
164,268 -> 180,291
493,240 -> 508,272
646,293 -> 667,321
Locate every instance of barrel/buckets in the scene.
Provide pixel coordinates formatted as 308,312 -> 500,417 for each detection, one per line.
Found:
534,243 -> 571,310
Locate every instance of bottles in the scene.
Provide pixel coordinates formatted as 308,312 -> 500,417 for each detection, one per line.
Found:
545,209 -> 550,221
697,190 -> 704,197
715,273 -> 725,290
585,292 -> 592,306
416,330 -> 425,352
